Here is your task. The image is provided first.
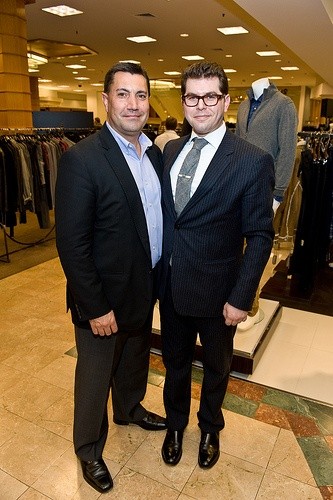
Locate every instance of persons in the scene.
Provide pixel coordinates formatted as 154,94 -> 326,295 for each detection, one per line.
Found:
237,77 -> 297,331
94,118 -> 102,126
154,117 -> 180,154
55,64 -> 164,493
159,61 -> 275,470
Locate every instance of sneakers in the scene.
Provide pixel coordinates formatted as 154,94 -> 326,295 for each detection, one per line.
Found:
236,309 -> 264,330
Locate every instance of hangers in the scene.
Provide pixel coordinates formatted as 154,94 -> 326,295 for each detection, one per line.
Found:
305,131 -> 333,163
1,127 -> 95,140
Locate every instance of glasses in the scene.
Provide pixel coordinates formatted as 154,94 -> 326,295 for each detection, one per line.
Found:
182,94 -> 224,107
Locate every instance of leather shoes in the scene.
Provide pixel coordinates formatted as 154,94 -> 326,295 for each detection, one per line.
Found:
80,460 -> 113,493
198,432 -> 220,469
162,428 -> 183,464
113,410 -> 166,431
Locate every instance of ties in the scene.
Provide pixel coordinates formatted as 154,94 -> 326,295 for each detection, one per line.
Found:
174,137 -> 208,217
